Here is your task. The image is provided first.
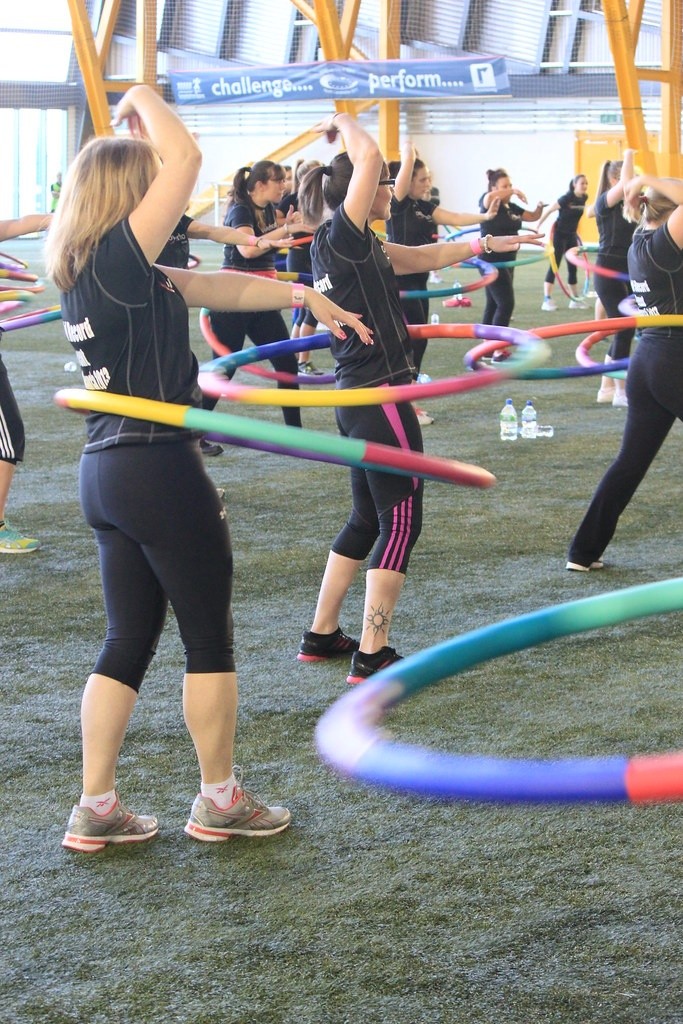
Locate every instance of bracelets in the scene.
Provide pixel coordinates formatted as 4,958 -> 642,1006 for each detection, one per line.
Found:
513,189 -> 519,194
249,235 -> 254,246
256,238 -> 262,247
284,223 -> 288,234
470,238 -> 482,255
484,234 -> 493,253
330,112 -> 344,130
291,283 -> 304,308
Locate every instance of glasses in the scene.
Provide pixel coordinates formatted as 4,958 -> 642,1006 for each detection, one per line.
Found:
374,178 -> 401,190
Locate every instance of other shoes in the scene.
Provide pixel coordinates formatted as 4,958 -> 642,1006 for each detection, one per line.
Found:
297,361 -> 326,376
564,557 -> 604,573
414,408 -> 437,427
482,350 -> 515,364
595,387 -> 615,403
199,441 -> 229,457
618,391 -> 631,408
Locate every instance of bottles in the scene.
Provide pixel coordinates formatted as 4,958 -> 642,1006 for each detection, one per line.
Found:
453,280 -> 462,300
64,361 -> 77,371
499,398 -> 518,441
521,400 -> 537,439
431,312 -> 439,324
417,373 -> 431,384
519,425 -> 554,438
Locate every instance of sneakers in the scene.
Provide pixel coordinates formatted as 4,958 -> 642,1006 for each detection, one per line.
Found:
60,793 -> 166,853
539,300 -> 559,312
569,299 -> 589,310
297,628 -> 363,661
346,646 -> 410,683
0,524 -> 46,554
182,765 -> 290,844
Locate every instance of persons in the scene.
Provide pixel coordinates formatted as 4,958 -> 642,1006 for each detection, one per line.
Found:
0,215 -> 59,554
296,112 -> 545,685
47,84 -> 374,853
153,142 -> 683,458
565,175 -> 683,572
